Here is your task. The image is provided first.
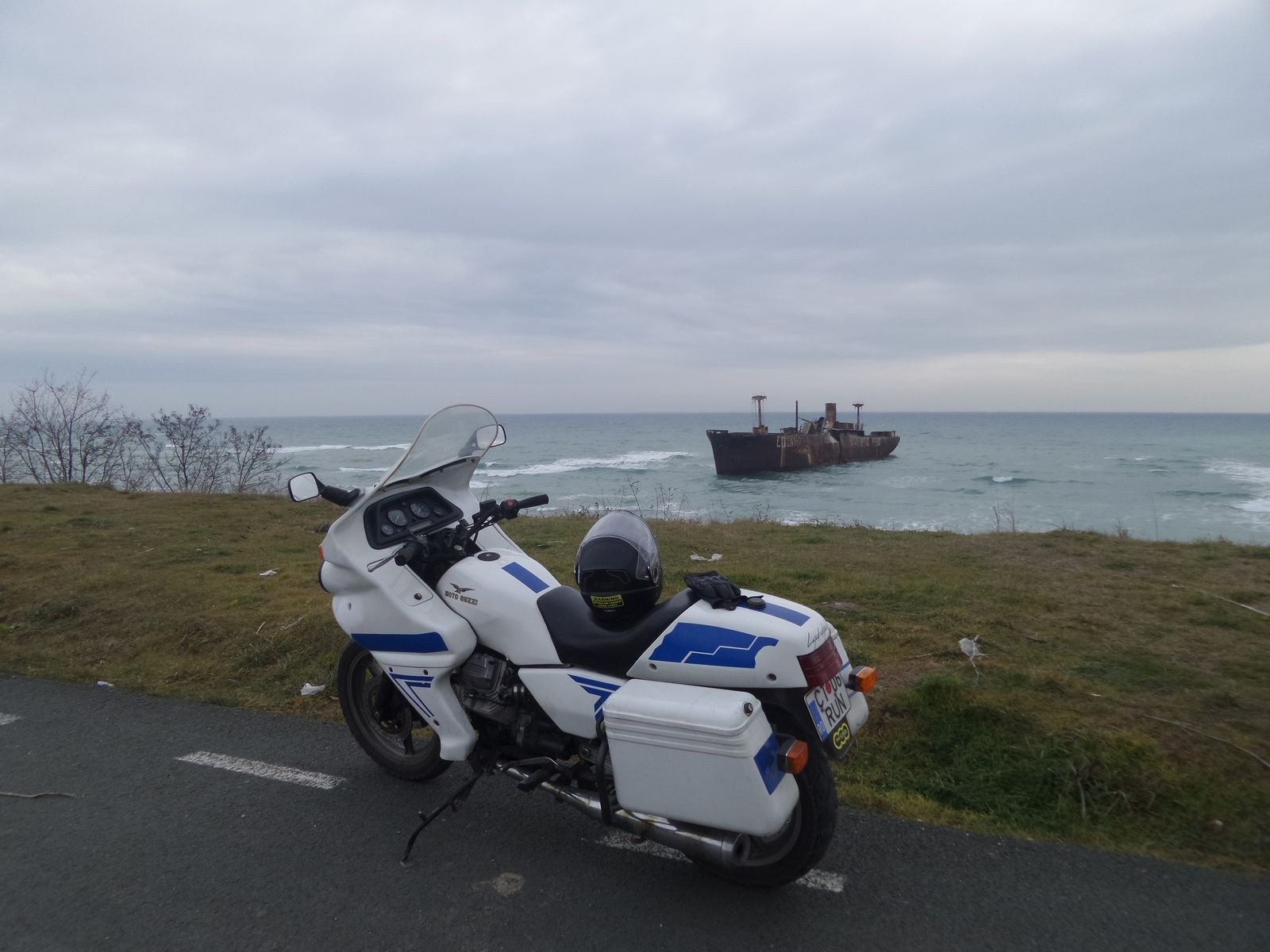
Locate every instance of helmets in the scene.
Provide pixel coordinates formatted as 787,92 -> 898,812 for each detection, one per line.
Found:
573,510 -> 662,617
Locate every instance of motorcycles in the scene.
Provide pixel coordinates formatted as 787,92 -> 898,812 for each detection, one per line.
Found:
283,401 -> 880,889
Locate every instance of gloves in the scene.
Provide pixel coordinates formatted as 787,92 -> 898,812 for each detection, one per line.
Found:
684,571 -> 764,610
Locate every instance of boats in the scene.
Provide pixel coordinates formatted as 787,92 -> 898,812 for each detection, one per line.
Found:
705,395 -> 901,475
